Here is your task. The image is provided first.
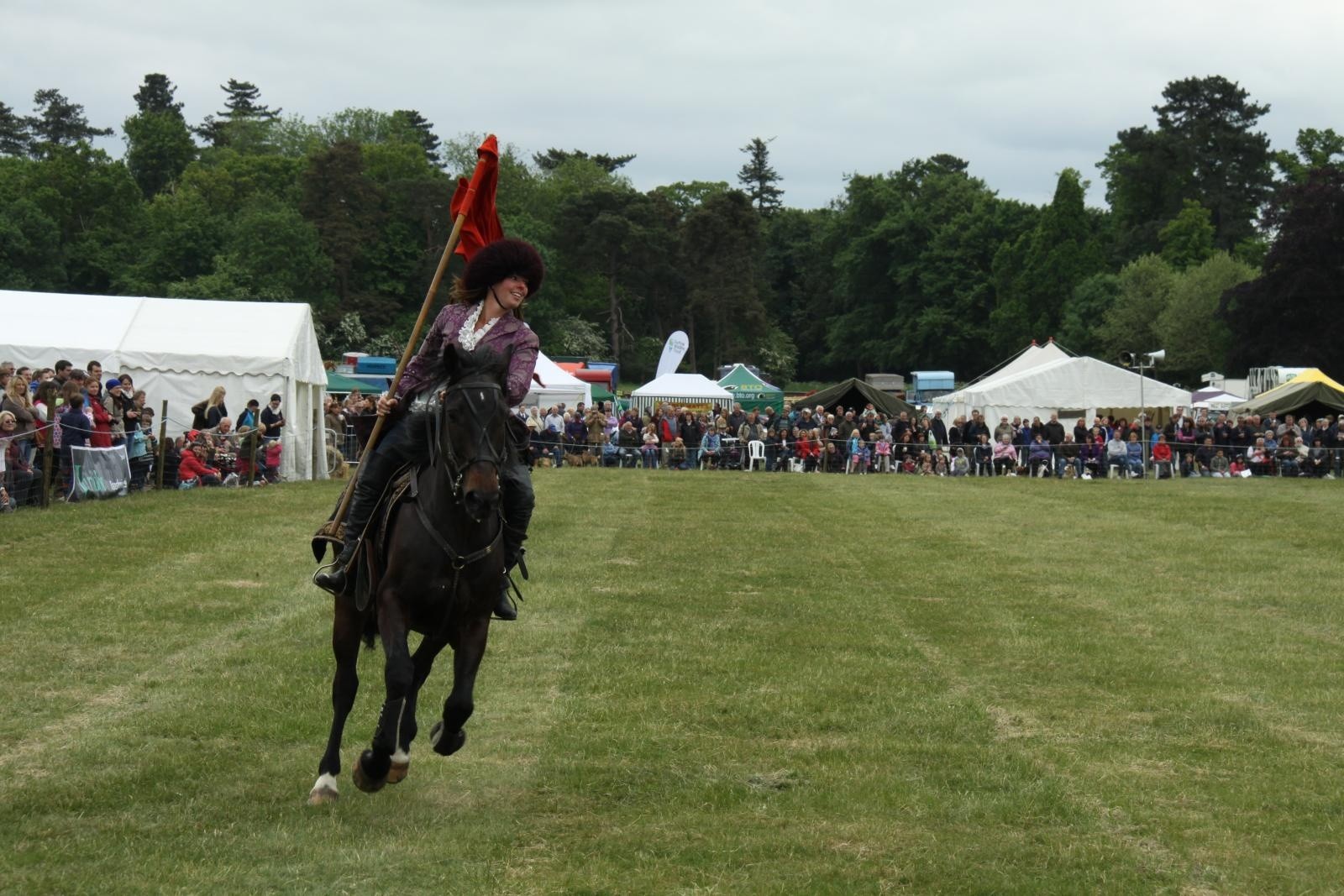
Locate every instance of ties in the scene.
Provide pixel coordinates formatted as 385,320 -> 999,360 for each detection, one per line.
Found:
522,415 -> 524,417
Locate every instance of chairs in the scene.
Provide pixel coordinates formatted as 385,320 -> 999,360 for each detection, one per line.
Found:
527,430 -> 1344,479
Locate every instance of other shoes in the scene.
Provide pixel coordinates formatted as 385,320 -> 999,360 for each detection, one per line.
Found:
1131,471 -> 1138,477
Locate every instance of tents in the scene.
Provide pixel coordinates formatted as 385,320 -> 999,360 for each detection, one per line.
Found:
932,356 -> 1196,478
323,372 -> 397,395
716,365 -> 785,418
792,378 -> 918,421
1230,368 -> 1344,419
0,287 -> 330,484
629,373 -> 735,420
508,350 -> 593,417
962,343 -> 1070,389
590,383 -> 618,417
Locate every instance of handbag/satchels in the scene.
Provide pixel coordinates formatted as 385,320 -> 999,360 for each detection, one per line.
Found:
1037,460 -> 1048,477
34,428 -> 45,445
928,430 -> 937,449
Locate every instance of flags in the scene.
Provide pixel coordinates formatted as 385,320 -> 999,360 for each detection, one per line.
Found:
449,133 -> 506,265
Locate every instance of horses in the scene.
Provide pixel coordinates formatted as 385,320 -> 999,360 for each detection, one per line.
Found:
308,343 -> 514,807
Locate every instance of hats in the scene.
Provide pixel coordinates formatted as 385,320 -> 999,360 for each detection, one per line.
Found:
719,424 -> 727,429
106,379 -> 121,391
591,406 -> 599,413
461,239 -> 543,294
528,423 -> 536,427
549,424 -> 556,431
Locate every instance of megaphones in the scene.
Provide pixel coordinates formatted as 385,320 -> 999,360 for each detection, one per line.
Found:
1143,349 -> 1166,362
1118,351 -> 1136,365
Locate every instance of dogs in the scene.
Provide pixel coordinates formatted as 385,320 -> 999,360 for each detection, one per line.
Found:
582,450 -> 600,466
564,453 -> 583,467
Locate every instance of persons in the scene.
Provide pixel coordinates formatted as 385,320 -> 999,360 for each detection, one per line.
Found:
312,239 -> 545,621
324,387 -> 1344,481
0,358 -> 287,514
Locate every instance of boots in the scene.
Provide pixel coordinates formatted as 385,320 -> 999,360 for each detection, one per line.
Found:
317,453 -> 393,596
493,505 -> 536,619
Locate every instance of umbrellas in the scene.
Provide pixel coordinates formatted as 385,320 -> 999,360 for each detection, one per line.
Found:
1191,386 -> 1248,404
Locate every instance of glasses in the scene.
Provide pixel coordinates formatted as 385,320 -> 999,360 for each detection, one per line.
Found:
5,368 -> 16,371
